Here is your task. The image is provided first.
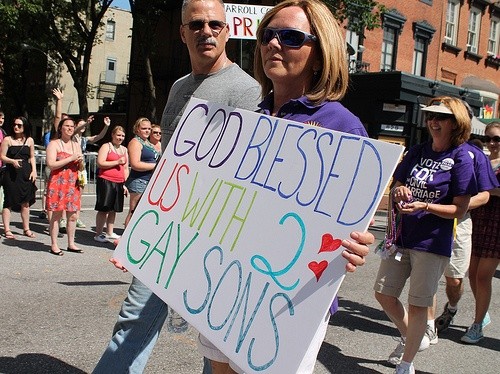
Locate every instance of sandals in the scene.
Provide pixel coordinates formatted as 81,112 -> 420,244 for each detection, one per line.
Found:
4,230 -> 15,239
24,230 -> 36,238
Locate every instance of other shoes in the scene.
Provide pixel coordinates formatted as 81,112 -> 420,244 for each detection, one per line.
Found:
67,247 -> 84,253
50,249 -> 63,256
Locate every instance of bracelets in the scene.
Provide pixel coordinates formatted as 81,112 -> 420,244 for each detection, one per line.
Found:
425,200 -> 430,212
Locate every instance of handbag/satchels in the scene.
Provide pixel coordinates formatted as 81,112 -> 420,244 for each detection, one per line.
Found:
78,161 -> 88,188
0,165 -> 6,186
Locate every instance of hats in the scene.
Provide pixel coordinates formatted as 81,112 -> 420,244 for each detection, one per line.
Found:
419,100 -> 454,114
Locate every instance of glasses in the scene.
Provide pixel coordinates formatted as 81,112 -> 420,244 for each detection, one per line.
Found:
260,27 -> 316,49
426,112 -> 450,120
483,136 -> 500,143
183,20 -> 226,30
153,131 -> 162,135
13,123 -> 23,128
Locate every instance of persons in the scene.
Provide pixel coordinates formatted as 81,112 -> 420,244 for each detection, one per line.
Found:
0,87 -> 165,257
92,0 -> 265,374
195,0 -> 377,374
372,95 -> 500,374
460,121 -> 500,345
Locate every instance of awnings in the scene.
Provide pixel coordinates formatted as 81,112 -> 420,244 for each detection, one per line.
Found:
470,114 -> 500,137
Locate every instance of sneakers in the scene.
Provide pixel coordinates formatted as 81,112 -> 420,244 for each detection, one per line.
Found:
59,217 -> 67,229
418,325 -> 438,351
459,322 -> 484,344
76,215 -> 86,228
396,360 -> 415,374
105,233 -> 121,239
482,312 -> 491,329
435,302 -> 457,333
94,234 -> 107,242
387,336 -> 407,365
